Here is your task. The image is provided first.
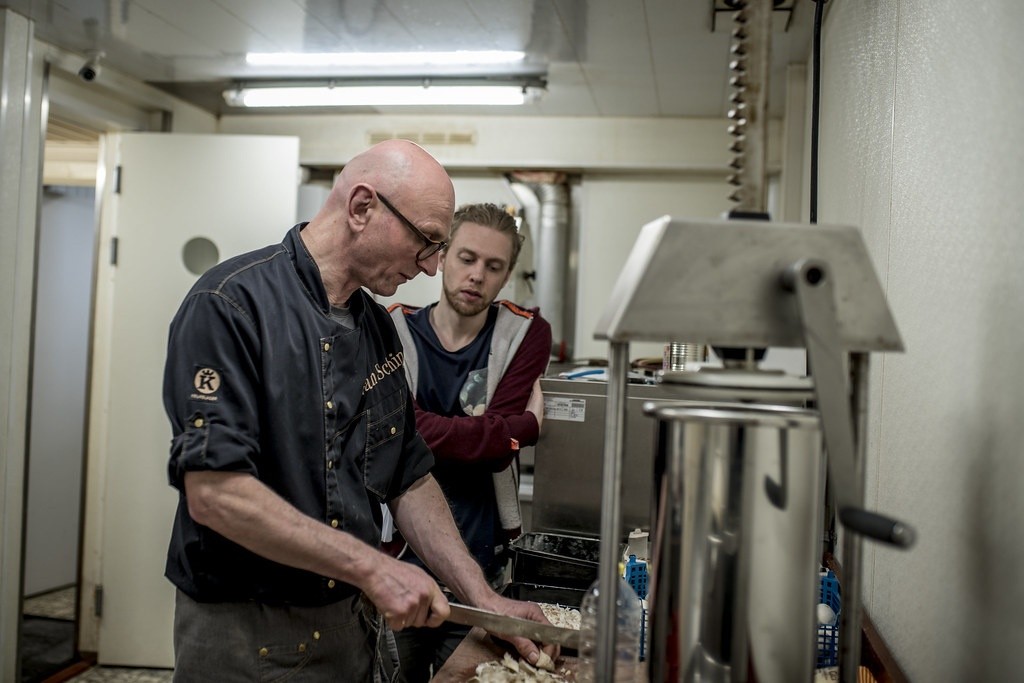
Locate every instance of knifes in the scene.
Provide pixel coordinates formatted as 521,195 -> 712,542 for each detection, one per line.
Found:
428,602 -> 585,648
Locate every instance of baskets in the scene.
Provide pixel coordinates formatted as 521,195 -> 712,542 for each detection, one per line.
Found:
623,554 -> 846,667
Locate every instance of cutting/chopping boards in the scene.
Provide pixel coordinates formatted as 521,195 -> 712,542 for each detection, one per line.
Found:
428,604 -> 647,683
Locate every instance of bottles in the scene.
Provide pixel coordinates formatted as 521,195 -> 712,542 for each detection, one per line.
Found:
579,565 -> 640,683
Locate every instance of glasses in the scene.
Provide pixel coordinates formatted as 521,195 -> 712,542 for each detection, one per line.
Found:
375,191 -> 448,262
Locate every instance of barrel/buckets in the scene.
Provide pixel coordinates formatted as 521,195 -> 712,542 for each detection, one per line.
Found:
646,401 -> 825,683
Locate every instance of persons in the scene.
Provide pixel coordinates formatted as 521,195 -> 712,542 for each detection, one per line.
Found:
374,203 -> 552,683
163,140 -> 561,683
